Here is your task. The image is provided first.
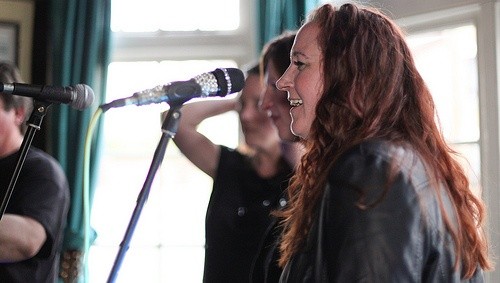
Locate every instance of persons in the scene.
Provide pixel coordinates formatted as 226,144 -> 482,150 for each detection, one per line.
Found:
160,63 -> 307,283
250,31 -> 310,283
276,3 -> 492,283
0,60 -> 70,283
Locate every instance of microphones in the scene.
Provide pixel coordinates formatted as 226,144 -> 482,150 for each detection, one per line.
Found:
0,82 -> 94,111
98,68 -> 245,113
